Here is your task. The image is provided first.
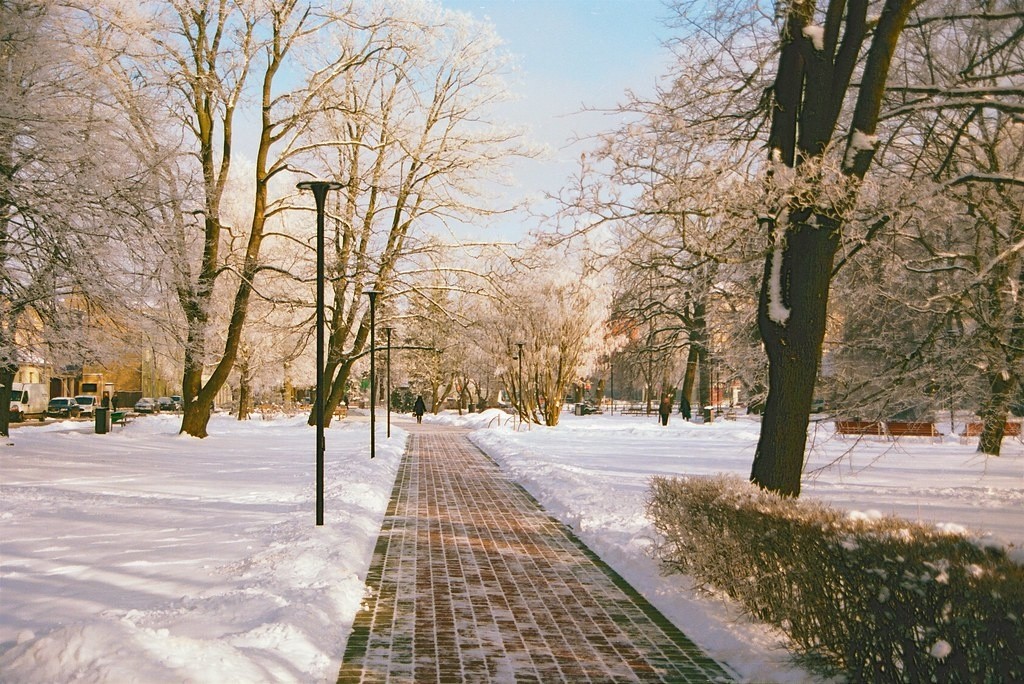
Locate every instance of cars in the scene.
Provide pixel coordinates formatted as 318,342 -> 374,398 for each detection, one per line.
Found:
48,396 -> 81,421
74,395 -> 100,418
133,397 -> 161,413
158,396 -> 182,412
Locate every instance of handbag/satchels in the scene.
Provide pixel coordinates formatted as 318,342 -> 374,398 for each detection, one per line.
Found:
412,411 -> 417,417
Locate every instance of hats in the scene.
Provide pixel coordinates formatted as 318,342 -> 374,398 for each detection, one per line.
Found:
664,398 -> 670,403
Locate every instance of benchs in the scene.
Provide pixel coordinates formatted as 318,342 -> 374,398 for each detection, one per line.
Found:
834,421 -> 885,438
959,422 -> 1022,445
886,422 -> 944,443
621,409 -> 659,417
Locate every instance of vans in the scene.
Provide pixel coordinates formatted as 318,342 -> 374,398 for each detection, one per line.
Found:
10,382 -> 49,423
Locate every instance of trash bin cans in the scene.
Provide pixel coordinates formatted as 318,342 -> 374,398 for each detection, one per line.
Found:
575,403 -> 586,416
468,402 -> 476,414
702,405 -> 715,423
94,407 -> 112,434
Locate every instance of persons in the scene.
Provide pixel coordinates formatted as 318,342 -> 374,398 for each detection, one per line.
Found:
658,398 -> 672,426
413,395 -> 427,424
111,392 -> 118,412
103,392 -> 109,409
679,397 -> 691,421
344,395 -> 350,409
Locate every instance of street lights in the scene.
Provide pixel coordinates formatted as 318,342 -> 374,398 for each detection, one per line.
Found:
609,362 -> 616,415
515,341 -> 527,422
295,178 -> 345,526
360,289 -> 385,460
384,326 -> 396,439
